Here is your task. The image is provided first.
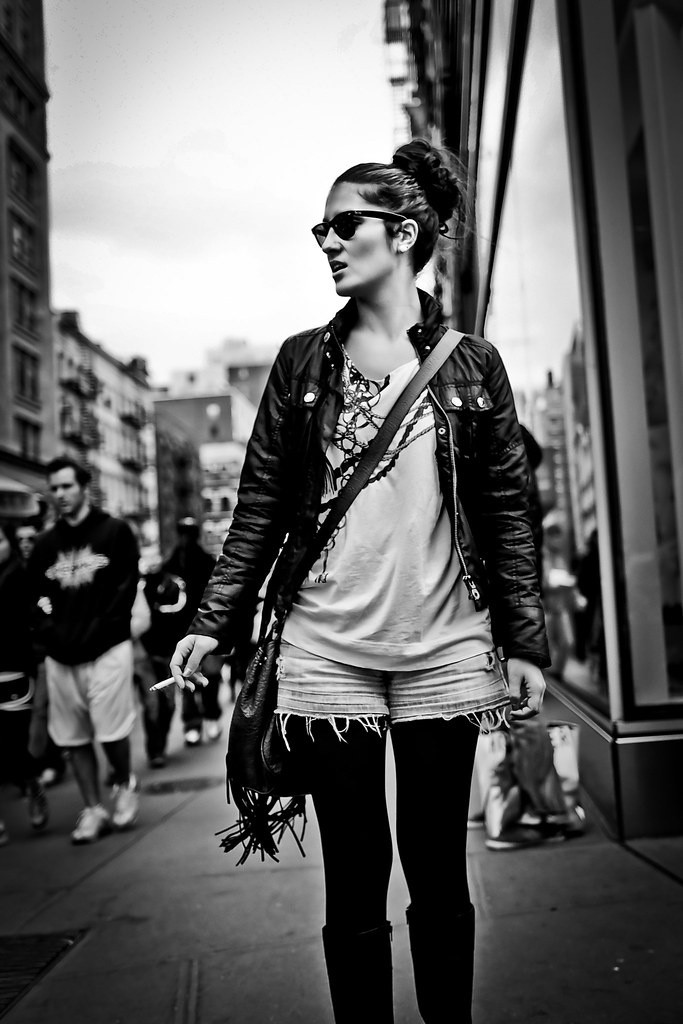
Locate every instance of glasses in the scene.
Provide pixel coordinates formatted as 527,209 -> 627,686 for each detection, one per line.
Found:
311,210 -> 407,248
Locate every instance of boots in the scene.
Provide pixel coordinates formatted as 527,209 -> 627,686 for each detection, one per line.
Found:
407,903 -> 475,1024
323,922 -> 394,1024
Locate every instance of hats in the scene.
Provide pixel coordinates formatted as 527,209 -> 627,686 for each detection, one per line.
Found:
177,513 -> 201,527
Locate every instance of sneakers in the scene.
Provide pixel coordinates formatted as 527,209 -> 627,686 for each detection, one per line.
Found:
109,772 -> 142,828
70,802 -> 110,845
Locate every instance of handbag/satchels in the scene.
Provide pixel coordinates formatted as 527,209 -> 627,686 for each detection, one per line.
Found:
214,635 -> 312,868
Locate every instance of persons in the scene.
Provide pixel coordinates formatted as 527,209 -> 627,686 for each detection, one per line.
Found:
170,140 -> 552,1024
25,456 -> 141,842
167,516 -> 221,743
16,525 -> 64,785
140,540 -> 186,766
0,523 -> 52,841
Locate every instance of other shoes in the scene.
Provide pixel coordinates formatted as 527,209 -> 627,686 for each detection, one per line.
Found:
485,827 -> 541,849
151,757 -> 167,768
29,788 -> 49,830
539,814 -> 597,843
206,723 -> 222,741
185,729 -> 201,746
0,821 -> 10,847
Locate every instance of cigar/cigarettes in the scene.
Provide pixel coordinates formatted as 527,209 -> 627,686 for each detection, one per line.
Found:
148,676 -> 174,691
